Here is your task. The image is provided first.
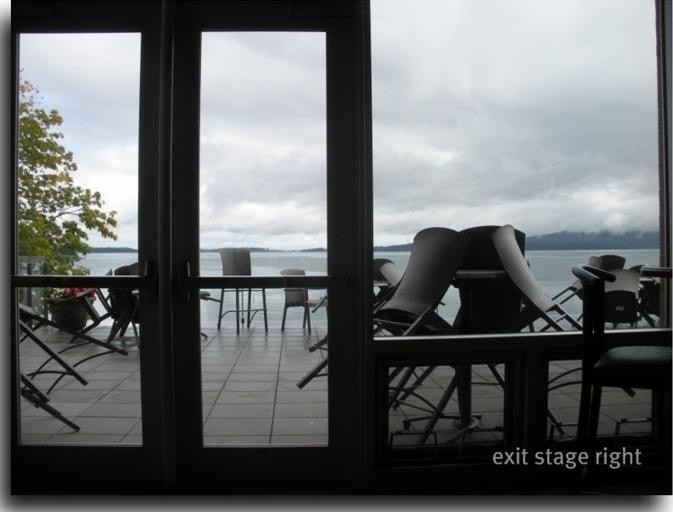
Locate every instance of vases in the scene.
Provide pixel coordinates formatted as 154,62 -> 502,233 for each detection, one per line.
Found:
43,297 -> 96,331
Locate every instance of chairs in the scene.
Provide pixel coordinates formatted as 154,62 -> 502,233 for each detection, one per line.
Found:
293,218 -> 673,469
279,267 -> 307,330
213,247 -> 270,335
65,261 -> 222,345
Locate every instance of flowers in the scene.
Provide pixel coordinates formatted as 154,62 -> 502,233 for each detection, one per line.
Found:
43,286 -> 95,299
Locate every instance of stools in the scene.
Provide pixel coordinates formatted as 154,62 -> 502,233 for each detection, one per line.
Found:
572,344 -> 673,495
